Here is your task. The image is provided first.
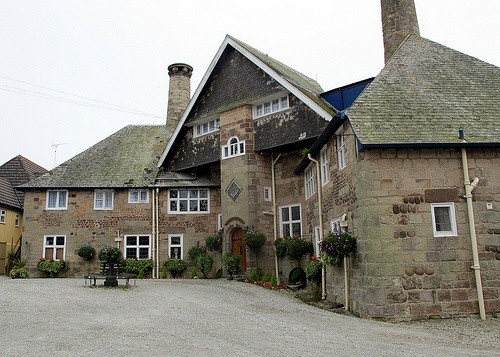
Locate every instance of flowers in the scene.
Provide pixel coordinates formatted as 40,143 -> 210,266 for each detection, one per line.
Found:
10,231 -> 357,288
